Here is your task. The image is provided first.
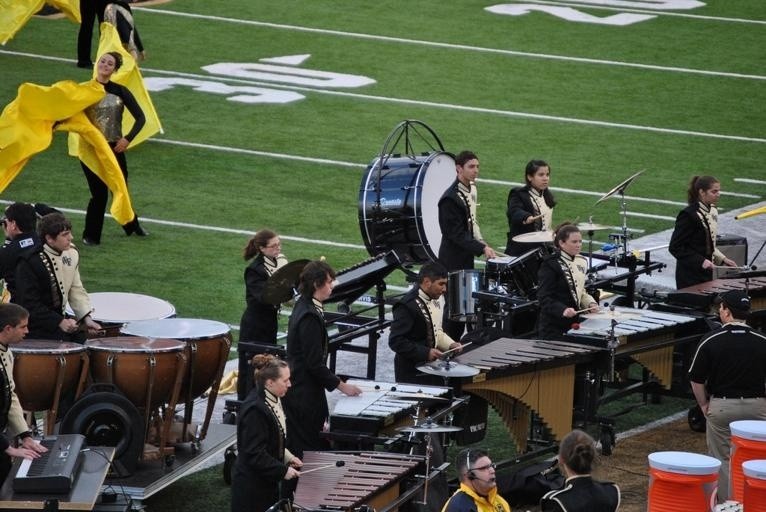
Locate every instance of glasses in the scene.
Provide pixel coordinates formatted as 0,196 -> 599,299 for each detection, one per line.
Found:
266,243 -> 281,248
470,463 -> 497,471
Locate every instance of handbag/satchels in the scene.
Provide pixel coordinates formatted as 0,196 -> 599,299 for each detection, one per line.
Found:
495,459 -> 566,504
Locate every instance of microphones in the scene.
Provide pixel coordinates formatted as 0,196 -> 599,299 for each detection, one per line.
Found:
476,476 -> 495,483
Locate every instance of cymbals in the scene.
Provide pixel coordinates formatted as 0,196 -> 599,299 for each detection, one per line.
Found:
386,395 -> 451,401
591,169 -> 647,208
415,359 -> 480,377
578,310 -> 641,319
512,231 -> 557,243
260,259 -> 312,303
395,424 -> 464,433
719,270 -> 766,279
574,222 -> 611,231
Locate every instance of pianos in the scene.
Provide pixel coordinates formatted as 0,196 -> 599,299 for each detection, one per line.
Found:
13,434 -> 86,495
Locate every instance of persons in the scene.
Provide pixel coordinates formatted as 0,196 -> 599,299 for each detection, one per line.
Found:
441,447 -> 512,512
229,353 -> 303,511
236,229 -> 288,401
283,260 -> 363,451
687,289 -> 766,502
668,174 -> 738,289
539,429 -> 621,511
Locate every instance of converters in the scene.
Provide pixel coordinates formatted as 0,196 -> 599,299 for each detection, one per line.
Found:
102,492 -> 117,503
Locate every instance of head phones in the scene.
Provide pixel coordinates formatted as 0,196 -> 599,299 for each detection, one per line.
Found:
465,448 -> 476,480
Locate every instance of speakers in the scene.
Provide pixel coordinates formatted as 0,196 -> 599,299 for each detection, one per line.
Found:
712,233 -> 747,280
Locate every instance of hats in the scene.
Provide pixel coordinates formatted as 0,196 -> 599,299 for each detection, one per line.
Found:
714,290 -> 750,315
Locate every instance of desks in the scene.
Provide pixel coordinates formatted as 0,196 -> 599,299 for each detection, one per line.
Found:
0,444 -> 118,511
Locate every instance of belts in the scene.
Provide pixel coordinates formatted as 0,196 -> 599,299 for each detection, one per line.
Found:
714,394 -> 762,399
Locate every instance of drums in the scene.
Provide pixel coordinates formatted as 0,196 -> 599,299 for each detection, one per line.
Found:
447,247 -> 544,324
5,292 -> 230,412
357,151 -> 456,264
